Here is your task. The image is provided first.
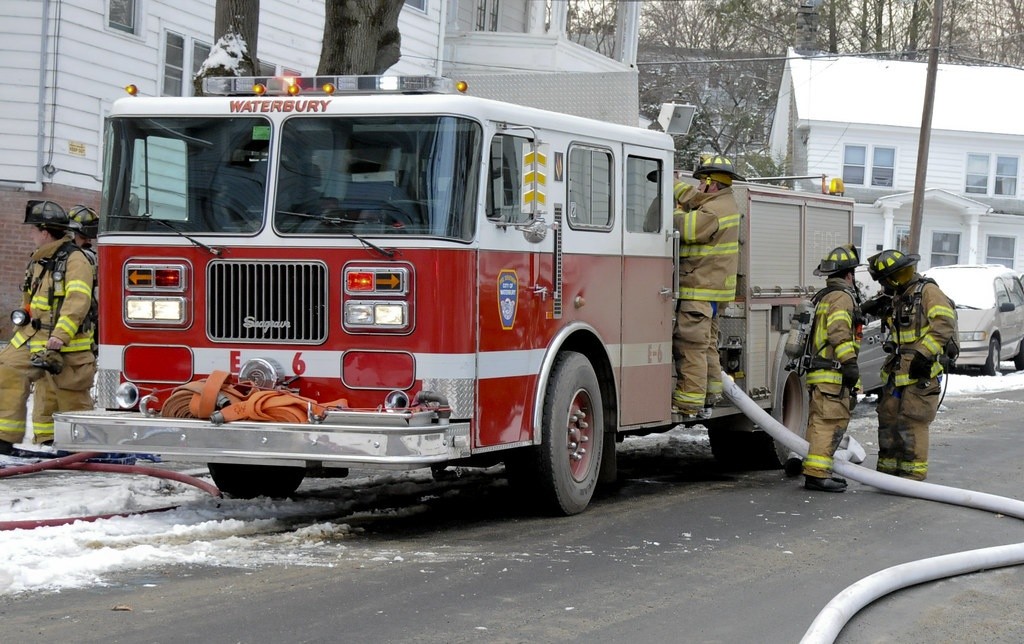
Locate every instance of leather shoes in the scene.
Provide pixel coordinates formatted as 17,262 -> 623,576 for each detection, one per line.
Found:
805,475 -> 847,492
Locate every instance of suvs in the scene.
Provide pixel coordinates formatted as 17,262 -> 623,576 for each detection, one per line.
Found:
921,263 -> 1024,376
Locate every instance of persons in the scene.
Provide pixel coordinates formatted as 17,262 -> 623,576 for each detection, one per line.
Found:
860,249 -> 956,480
672,156 -> 741,417
803,245 -> 860,491
0,199 -> 99,456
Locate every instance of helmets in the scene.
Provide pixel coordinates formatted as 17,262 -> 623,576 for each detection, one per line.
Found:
22,200 -> 71,228
692,155 -> 747,185
813,246 -> 862,276
867,249 -> 920,281
65,203 -> 99,238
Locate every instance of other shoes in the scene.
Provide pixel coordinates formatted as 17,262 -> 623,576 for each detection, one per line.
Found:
671,403 -> 705,418
706,393 -> 723,407
0,439 -> 13,454
892,468 -> 916,479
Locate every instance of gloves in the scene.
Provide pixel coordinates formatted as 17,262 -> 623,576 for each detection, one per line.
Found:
908,352 -> 932,379
840,358 -> 859,388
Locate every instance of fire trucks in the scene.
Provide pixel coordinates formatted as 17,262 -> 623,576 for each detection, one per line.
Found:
47,72 -> 894,518
